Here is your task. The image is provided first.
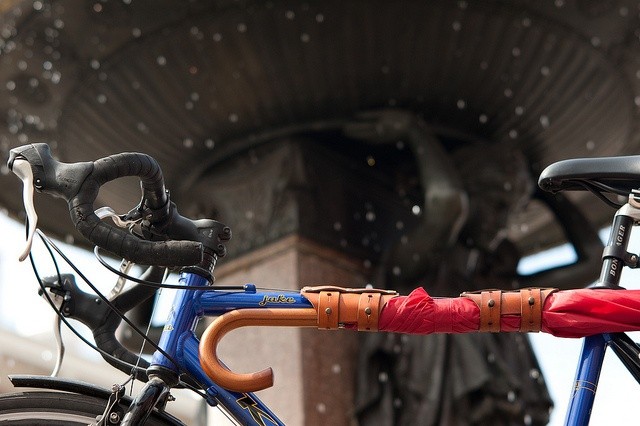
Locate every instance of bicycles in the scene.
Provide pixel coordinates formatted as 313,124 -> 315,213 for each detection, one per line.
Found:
0,142 -> 640,425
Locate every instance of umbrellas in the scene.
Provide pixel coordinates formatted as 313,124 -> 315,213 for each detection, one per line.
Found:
199,286 -> 639,392
